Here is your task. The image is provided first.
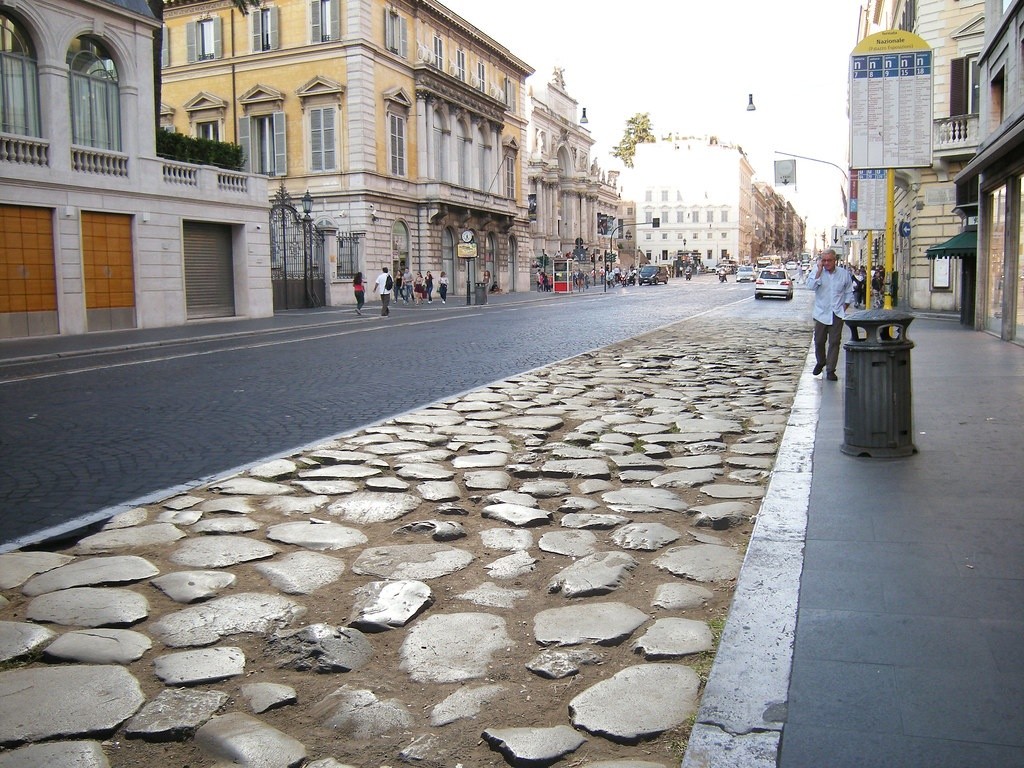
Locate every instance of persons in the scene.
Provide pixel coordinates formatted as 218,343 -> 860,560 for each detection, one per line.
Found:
621,268 -> 634,277
373,267 -> 394,316
536,269 -> 552,292
573,269 -> 590,289
591,269 -> 596,285
393,268 -> 433,305
796,263 -> 805,283
438,271 -> 449,304
483,270 -> 490,304
607,272 -> 615,288
606,265 -> 609,271
848,262 -> 885,307
614,266 -> 620,283
353,272 -> 365,315
720,267 -> 727,281
599,266 -> 605,284
697,265 -> 700,274
805,249 -> 853,380
686,266 -> 692,272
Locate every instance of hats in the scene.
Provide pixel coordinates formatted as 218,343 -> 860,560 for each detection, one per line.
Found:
494,281 -> 497,283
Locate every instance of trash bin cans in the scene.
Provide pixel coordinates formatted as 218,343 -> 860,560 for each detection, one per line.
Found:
839,309 -> 920,459
474,281 -> 487,305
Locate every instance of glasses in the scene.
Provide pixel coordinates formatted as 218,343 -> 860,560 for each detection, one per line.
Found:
417,274 -> 419,275
821,258 -> 835,263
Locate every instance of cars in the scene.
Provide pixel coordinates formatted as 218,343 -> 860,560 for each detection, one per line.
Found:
716,264 -> 735,275
736,266 -> 756,282
756,254 -> 813,269
755,268 -> 795,300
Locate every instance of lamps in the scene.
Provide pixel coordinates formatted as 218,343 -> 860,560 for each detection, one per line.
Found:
580,108 -> 588,123
746,93 -> 756,111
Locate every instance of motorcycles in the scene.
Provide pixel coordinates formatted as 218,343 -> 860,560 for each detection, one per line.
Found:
719,271 -> 727,282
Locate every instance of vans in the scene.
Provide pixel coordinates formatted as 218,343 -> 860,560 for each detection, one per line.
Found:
639,265 -> 668,285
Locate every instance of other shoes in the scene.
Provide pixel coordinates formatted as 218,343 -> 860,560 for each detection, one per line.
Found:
394,301 -> 397,304
355,309 -> 362,315
827,372 -> 838,381
813,360 -> 827,375
441,298 -> 445,304
421,300 -> 424,304
428,300 -> 433,303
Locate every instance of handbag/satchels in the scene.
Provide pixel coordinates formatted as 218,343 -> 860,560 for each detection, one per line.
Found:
385,275 -> 392,291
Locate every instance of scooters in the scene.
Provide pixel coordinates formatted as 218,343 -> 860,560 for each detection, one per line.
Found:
626,276 -> 635,286
685,270 -> 691,280
620,274 -> 626,286
